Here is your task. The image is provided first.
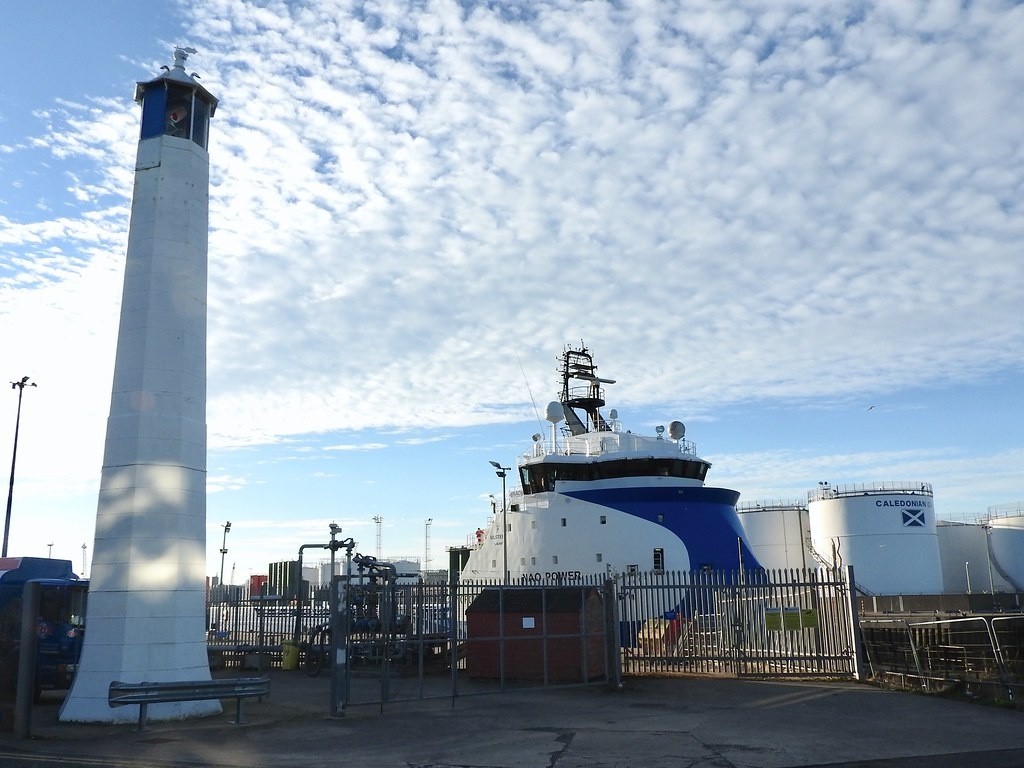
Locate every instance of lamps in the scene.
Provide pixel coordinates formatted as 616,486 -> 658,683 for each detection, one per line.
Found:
531,433 -> 542,442
655,425 -> 665,436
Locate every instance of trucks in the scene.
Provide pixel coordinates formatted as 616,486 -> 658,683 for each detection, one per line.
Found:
0,555 -> 91,689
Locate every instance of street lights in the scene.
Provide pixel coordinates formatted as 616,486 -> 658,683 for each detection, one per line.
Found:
220,520 -> 232,586
0,375 -> 39,557
966,560 -> 971,594
488,455 -> 512,585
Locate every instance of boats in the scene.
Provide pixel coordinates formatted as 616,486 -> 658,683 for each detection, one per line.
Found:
458,338 -> 777,648
